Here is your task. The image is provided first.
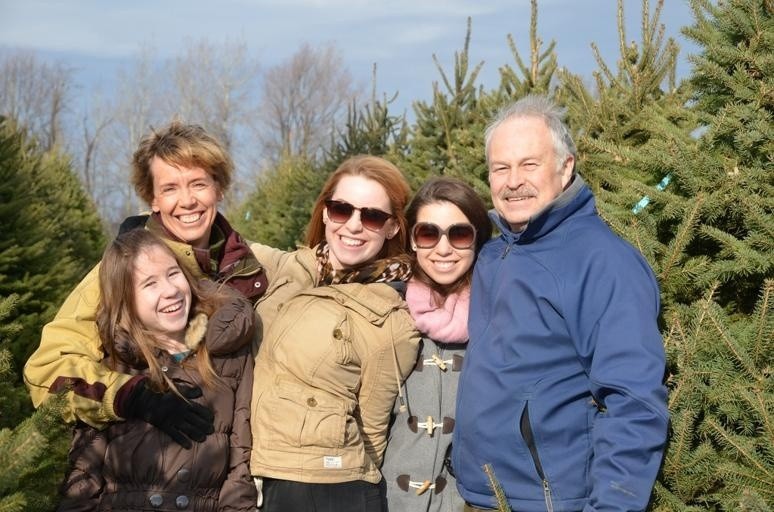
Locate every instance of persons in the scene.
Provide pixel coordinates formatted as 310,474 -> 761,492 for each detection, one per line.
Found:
23,121 -> 269,450
450,91 -> 670,512
380,178 -> 494,512
54,227 -> 258,511
241,155 -> 423,510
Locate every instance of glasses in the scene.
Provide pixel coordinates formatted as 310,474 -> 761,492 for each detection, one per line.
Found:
413,222 -> 476,250
322,199 -> 394,233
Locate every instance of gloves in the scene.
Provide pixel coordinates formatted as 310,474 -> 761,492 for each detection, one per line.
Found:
126,378 -> 215,448
